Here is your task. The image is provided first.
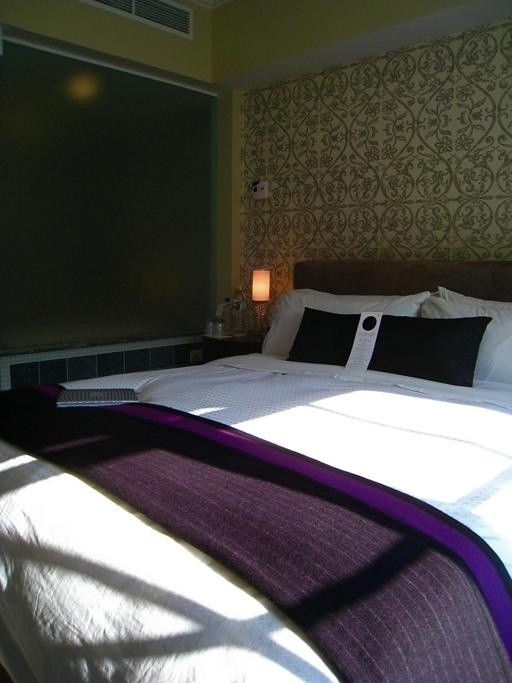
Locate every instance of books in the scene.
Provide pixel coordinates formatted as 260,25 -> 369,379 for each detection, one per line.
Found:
55,387 -> 140,408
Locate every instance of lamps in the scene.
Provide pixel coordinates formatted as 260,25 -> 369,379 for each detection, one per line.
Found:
251,269 -> 271,304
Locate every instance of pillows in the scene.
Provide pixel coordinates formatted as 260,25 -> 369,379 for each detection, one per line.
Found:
260,283 -> 510,388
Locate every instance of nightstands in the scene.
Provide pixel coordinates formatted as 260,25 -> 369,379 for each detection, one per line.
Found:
202,334 -> 266,364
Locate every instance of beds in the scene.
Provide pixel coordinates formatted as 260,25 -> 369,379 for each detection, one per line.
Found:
0,256 -> 512,682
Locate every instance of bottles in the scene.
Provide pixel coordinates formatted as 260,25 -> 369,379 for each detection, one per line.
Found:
206,320 -> 225,337
221,297 -> 234,335
